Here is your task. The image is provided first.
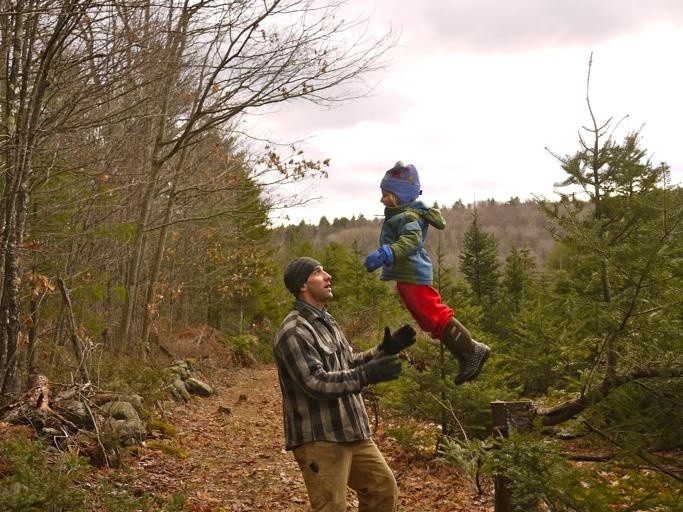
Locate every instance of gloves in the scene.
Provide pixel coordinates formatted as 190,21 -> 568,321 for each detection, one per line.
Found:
377,323 -> 417,357
363,244 -> 394,273
359,350 -> 402,387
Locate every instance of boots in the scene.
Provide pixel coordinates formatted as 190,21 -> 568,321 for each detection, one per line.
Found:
442,316 -> 491,382
445,344 -> 466,385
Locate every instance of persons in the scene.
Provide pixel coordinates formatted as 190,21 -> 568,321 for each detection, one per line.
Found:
272,256 -> 415,512
364,160 -> 491,385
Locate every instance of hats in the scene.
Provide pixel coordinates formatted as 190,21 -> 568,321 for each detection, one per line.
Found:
283,257 -> 322,295
380,160 -> 423,203
